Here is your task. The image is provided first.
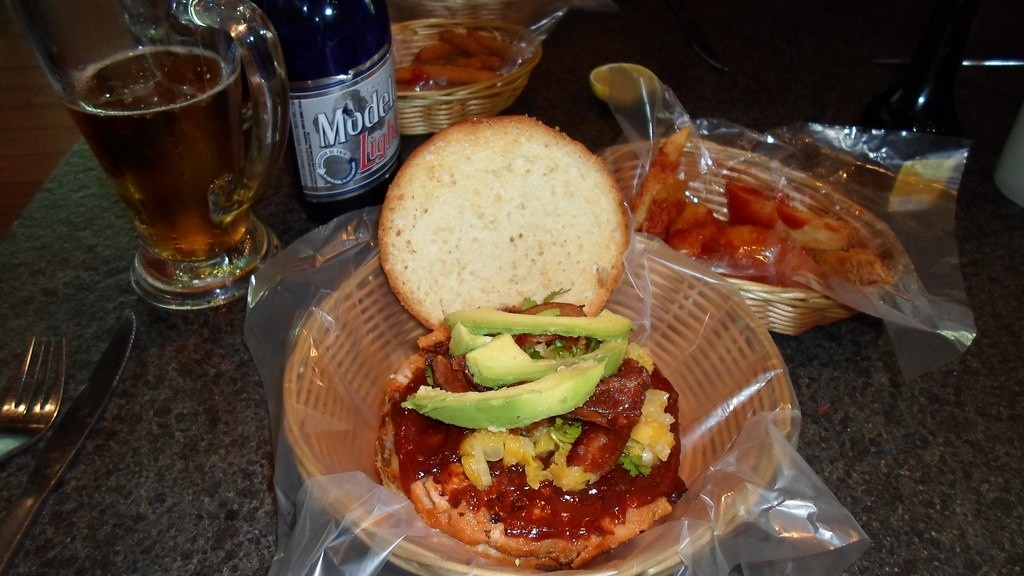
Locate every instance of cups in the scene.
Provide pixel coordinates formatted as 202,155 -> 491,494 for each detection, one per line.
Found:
9,0 -> 290,310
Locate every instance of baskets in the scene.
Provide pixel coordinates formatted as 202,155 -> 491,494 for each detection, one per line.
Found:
590,137 -> 905,338
391,15 -> 542,136
403,0 -> 543,28
281,252 -> 794,575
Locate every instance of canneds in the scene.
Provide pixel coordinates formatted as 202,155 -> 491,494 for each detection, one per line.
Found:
253,1 -> 402,210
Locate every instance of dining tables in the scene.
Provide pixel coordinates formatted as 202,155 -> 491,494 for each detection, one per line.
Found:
1,26 -> 1024,576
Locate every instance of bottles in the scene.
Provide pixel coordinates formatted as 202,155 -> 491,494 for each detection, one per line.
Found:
242,0 -> 399,225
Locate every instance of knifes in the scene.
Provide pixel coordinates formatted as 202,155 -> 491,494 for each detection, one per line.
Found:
0,309 -> 134,574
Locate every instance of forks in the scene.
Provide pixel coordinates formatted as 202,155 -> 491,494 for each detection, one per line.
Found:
0,335 -> 66,460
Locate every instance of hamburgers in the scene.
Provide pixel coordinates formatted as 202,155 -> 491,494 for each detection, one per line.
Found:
376,111 -> 683,571
